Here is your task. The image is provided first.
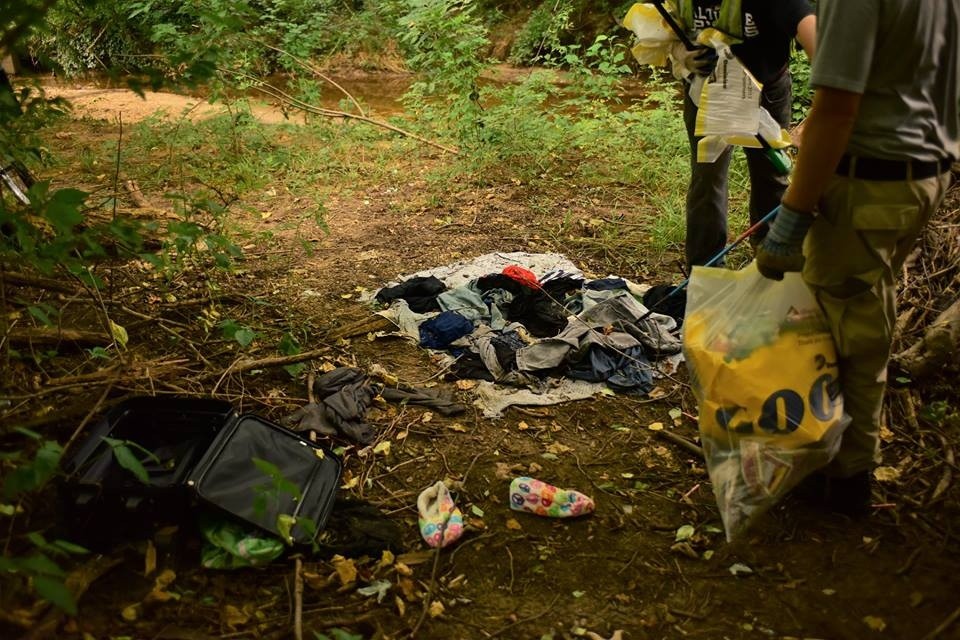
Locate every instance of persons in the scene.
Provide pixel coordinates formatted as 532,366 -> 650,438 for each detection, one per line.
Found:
664,0 -> 819,282
752,0 -> 960,513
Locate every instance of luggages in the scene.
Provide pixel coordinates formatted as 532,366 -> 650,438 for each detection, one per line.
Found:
57,392 -> 344,545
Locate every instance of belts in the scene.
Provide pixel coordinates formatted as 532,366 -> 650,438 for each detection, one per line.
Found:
837,156 -> 956,181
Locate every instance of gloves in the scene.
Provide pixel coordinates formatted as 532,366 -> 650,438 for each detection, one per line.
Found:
675,38 -> 718,76
757,207 -> 815,280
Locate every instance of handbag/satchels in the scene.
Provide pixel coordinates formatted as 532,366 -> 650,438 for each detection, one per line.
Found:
682,259 -> 855,541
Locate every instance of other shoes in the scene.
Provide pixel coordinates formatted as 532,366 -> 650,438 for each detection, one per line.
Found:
416,482 -> 466,548
509,479 -> 596,518
805,468 -> 872,518
